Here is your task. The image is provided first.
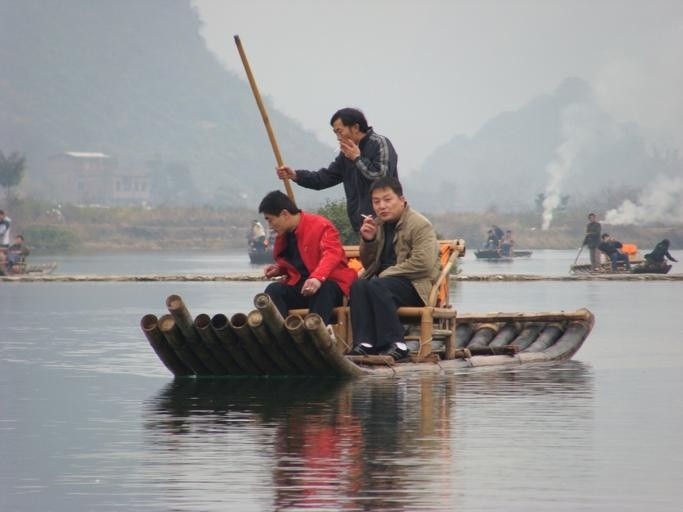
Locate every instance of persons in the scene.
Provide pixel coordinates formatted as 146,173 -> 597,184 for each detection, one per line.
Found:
275,107 -> 398,240
0,208 -> 31,280
598,233 -> 631,272
581,213 -> 601,271
244,218 -> 278,257
345,176 -> 443,360
257,190 -> 358,328
644,238 -> 679,266
483,224 -> 514,257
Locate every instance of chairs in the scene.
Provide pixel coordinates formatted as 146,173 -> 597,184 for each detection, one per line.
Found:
0,246 -> 34,275
486,239 -> 515,250
286,239 -> 465,359
602,249 -> 630,273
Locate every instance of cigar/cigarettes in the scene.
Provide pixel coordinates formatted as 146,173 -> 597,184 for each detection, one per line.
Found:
360,213 -> 370,220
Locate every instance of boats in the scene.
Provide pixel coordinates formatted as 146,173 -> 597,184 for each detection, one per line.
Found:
0,248 -> 57,275
139,238 -> 595,379
248,252 -> 276,264
569,244 -> 672,274
474,240 -> 533,258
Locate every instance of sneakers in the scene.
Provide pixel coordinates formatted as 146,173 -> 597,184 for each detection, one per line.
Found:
346,342 -> 378,355
380,343 -> 409,362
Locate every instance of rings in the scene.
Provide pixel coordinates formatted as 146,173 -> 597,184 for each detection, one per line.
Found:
304,287 -> 311,291
